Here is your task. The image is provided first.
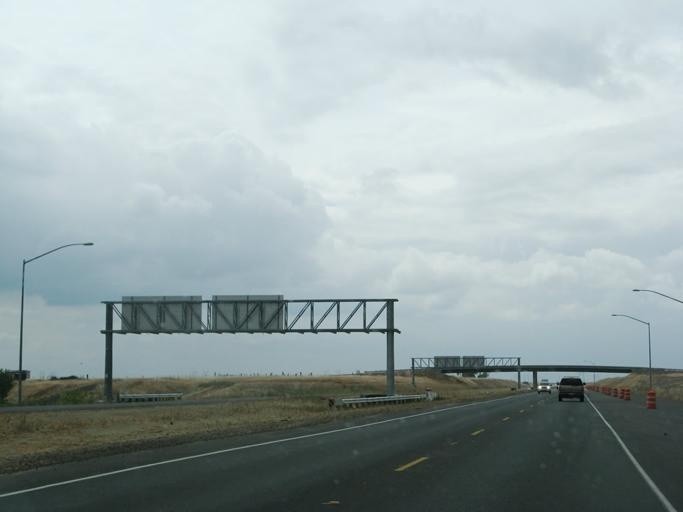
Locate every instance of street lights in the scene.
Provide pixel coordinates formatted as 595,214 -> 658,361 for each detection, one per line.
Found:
610,313 -> 653,389
632,289 -> 683,303
18,242 -> 94,406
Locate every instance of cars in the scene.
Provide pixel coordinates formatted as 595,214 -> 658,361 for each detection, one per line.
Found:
556,377 -> 586,402
537,382 -> 553,395
